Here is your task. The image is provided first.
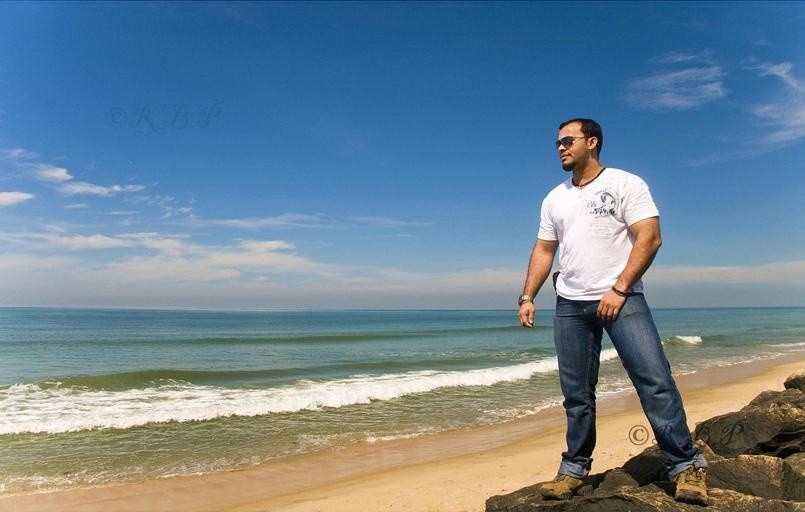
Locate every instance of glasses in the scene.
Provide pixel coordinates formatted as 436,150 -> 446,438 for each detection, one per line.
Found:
556,136 -> 584,148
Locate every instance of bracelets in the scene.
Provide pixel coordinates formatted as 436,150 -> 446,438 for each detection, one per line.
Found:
517,295 -> 534,306
612,286 -> 627,297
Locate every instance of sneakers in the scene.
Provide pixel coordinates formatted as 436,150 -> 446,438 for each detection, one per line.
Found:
537,474 -> 592,499
675,466 -> 707,505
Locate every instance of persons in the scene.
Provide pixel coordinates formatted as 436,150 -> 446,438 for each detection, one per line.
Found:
516,118 -> 713,506
